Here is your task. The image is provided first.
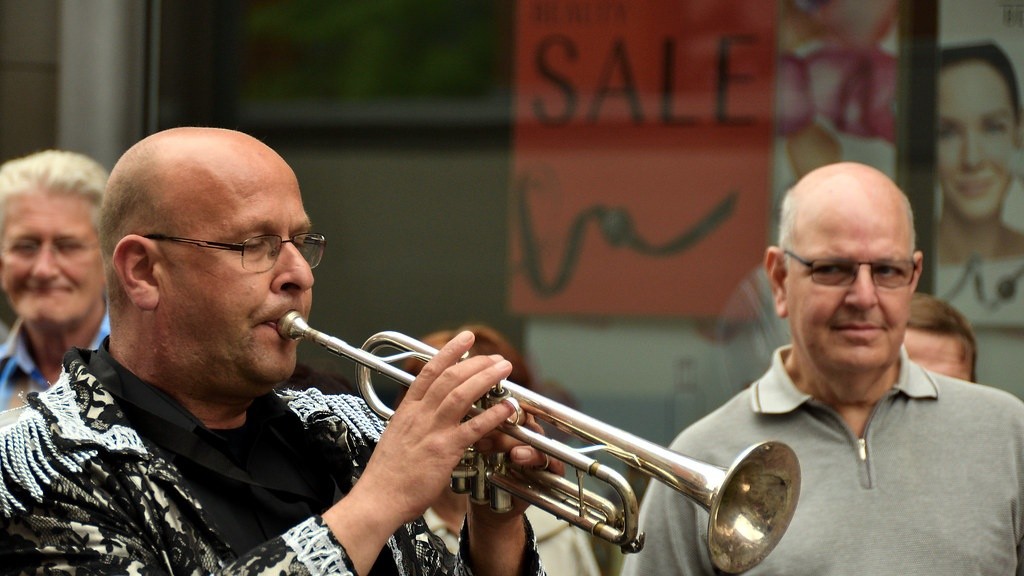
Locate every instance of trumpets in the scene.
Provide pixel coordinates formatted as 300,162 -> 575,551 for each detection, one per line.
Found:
276,307 -> 803,576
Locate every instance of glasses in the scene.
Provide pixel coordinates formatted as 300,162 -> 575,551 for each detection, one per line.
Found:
141,229 -> 329,273
781,248 -> 917,289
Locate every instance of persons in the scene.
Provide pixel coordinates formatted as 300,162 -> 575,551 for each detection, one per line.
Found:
0,127 -> 602,576
937,41 -> 1024,260
620,162 -> 1024,576
